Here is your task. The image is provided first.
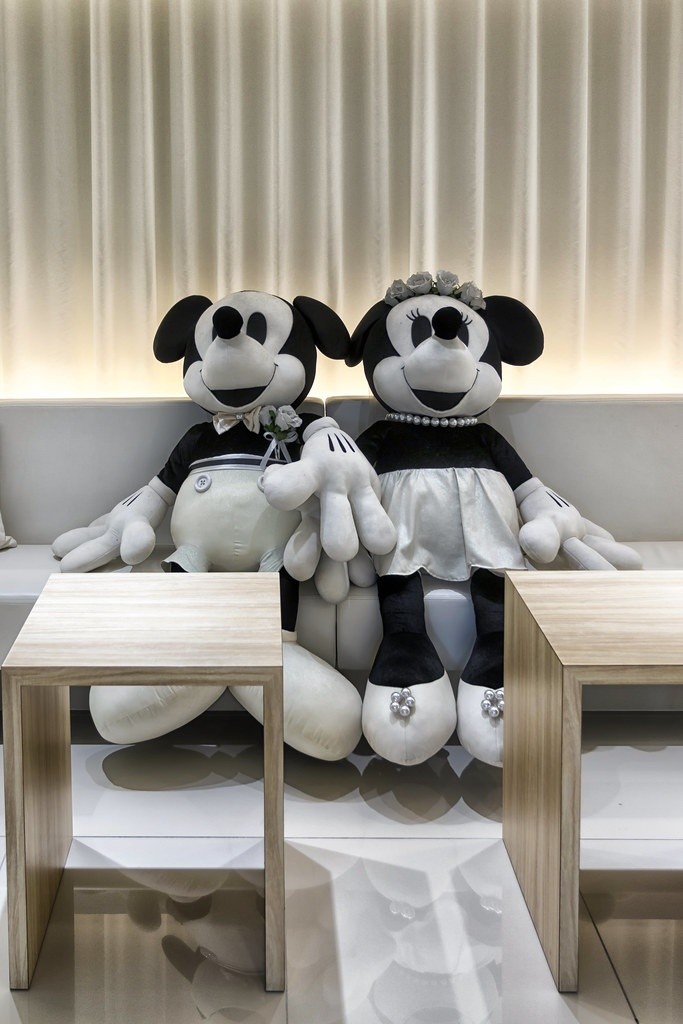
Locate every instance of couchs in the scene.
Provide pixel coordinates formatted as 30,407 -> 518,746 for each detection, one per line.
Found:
0,396 -> 683,710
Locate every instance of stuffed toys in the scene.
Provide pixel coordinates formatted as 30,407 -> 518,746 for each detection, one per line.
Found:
263,270 -> 643,769
52,289 -> 396,762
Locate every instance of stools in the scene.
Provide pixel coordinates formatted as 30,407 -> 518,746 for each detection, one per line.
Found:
1,574 -> 284,992
503,569 -> 683,991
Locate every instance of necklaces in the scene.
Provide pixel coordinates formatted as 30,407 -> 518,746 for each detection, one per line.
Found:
385,413 -> 478,426
